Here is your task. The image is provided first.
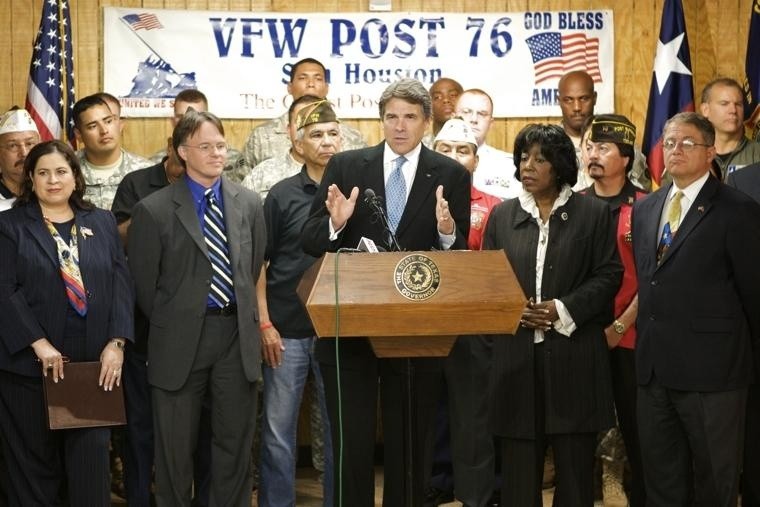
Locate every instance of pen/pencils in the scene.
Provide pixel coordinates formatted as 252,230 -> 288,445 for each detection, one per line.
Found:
35,356 -> 71,363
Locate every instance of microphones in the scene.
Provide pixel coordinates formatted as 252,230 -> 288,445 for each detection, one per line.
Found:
342,245 -> 387,252
364,188 -> 402,252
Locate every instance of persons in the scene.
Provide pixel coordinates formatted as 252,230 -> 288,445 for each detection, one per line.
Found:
2,59 -> 759,507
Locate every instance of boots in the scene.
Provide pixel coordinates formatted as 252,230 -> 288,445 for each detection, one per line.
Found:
602,459 -> 629,506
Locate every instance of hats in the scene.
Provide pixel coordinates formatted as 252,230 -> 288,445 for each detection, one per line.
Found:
0,105 -> 40,136
296,100 -> 340,130
588,114 -> 636,147
433,117 -> 479,155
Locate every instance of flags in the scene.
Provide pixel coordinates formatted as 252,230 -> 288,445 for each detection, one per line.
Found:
121,11 -> 165,32
525,31 -> 604,85
24,0 -> 79,157
640,0 -> 697,188
741,0 -> 760,125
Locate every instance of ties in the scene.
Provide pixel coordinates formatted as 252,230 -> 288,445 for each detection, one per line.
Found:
655,192 -> 685,268
204,188 -> 234,311
385,156 -> 408,246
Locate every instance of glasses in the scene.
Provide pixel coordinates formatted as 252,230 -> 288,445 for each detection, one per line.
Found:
662,138 -> 712,151
1,139 -> 36,152
182,143 -> 228,152
456,107 -> 492,119
